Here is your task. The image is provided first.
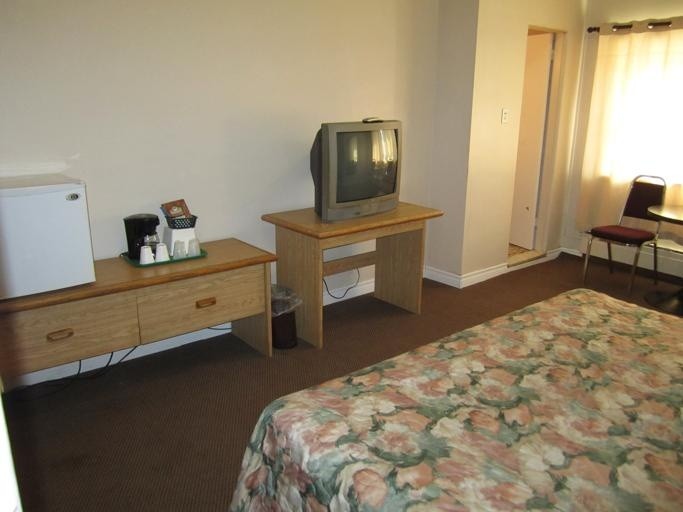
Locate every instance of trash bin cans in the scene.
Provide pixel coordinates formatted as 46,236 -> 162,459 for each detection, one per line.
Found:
271,284 -> 297,349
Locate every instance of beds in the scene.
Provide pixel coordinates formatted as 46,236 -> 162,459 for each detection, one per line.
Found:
228,286 -> 683,512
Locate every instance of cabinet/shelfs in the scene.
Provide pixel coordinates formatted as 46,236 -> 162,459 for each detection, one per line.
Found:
0,238 -> 278,413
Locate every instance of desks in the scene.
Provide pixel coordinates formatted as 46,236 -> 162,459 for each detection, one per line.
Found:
260,202 -> 444,349
648,203 -> 683,226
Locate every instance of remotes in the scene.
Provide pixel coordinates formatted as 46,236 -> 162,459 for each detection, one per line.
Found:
362,116 -> 384,122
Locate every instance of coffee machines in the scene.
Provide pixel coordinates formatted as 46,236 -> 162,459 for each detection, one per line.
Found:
121,211 -> 162,260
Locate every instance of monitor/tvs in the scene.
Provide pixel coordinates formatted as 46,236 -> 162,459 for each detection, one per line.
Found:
309,120 -> 402,223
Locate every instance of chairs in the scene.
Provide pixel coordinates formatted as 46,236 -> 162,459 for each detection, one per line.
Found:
584,174 -> 667,295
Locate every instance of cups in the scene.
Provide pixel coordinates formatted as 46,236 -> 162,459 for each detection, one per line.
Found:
139,239 -> 202,264
